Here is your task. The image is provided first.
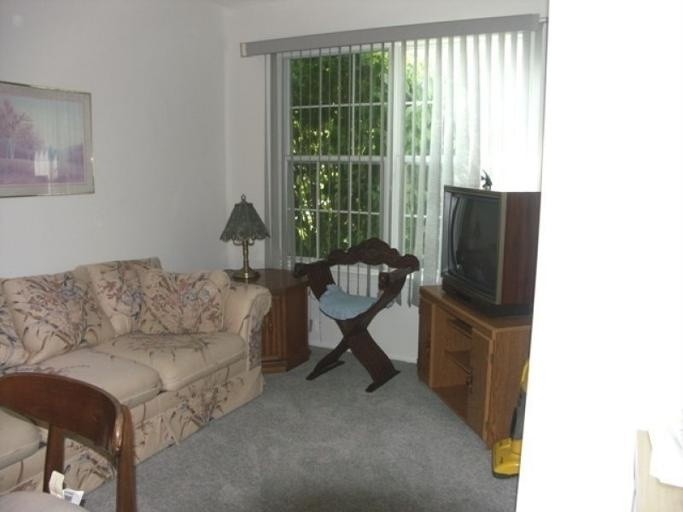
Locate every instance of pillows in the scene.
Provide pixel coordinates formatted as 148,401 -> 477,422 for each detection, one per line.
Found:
134,265 -> 228,336
1,256 -> 163,369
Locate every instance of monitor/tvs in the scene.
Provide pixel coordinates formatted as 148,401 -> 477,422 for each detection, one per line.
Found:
439,188 -> 541,318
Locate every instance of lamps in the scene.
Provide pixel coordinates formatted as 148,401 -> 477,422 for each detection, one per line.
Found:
217,195 -> 272,281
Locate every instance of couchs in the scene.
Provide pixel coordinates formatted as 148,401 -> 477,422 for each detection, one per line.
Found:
1,258 -> 274,503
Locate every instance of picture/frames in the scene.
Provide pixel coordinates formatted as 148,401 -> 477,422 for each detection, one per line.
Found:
1,81 -> 97,199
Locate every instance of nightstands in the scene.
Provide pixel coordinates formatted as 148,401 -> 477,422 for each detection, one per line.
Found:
223,269 -> 313,376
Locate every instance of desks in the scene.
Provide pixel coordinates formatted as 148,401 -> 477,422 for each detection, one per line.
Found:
416,285 -> 533,451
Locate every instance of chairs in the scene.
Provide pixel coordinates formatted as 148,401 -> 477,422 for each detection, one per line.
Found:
293,237 -> 422,394
1,373 -> 138,512
